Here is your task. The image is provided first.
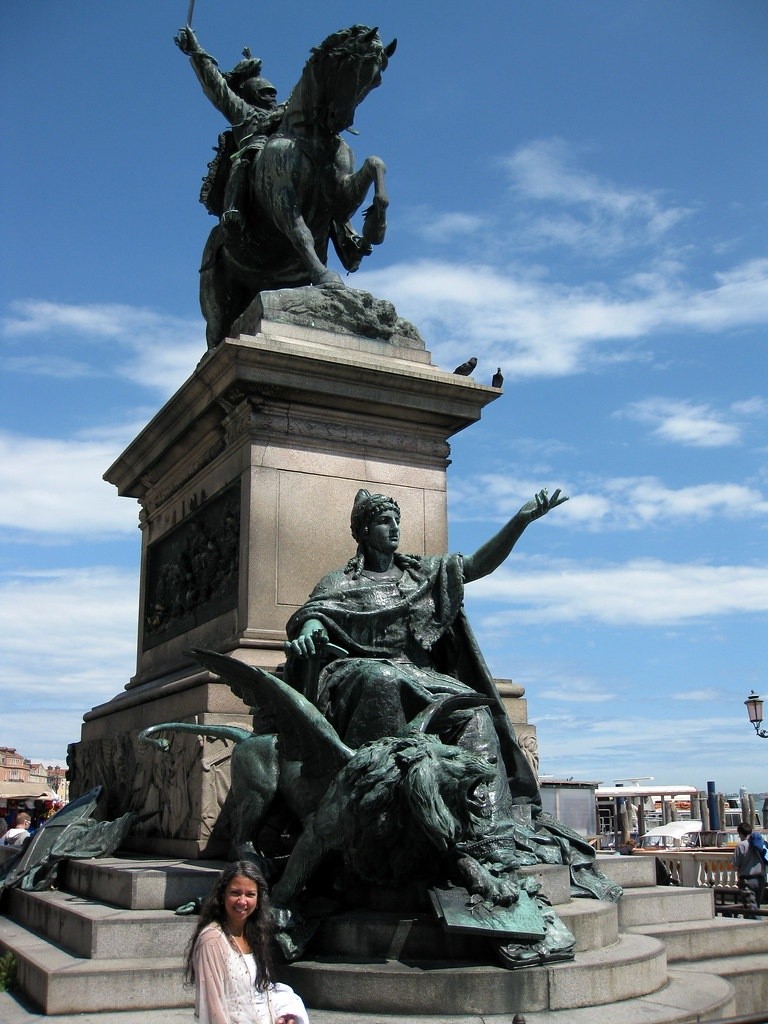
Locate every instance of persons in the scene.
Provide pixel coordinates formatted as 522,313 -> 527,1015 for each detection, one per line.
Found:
0,800 -> 63,849
182,860 -> 310,1024
284,489 -> 570,905
174,24 -> 373,273
630,803 -> 638,831
731,823 -> 768,920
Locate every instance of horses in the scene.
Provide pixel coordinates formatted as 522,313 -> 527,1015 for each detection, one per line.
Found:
199,24 -> 398,349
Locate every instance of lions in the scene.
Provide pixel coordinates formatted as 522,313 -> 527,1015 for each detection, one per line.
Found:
269,735 -> 520,960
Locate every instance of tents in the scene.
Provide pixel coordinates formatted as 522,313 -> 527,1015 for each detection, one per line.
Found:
0,781 -> 61,808
